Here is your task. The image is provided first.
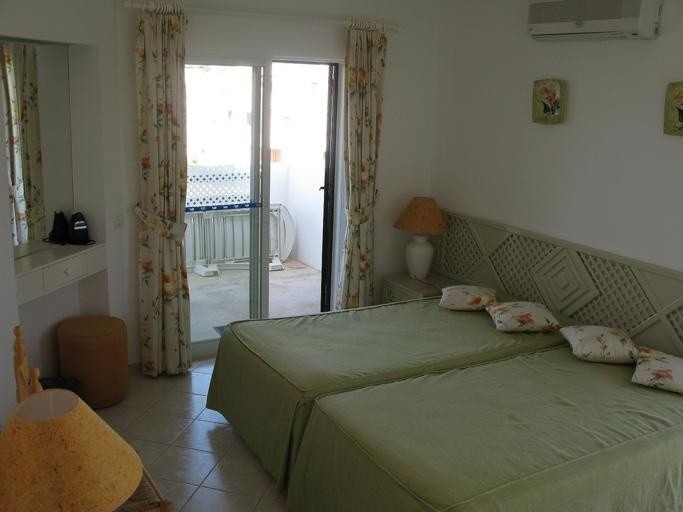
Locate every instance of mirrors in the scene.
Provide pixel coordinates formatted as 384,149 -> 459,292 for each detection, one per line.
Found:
0,35 -> 76,260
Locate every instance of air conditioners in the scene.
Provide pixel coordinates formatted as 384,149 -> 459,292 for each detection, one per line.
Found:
525,0 -> 663,43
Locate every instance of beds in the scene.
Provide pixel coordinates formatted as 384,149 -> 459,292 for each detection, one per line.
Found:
203,285 -> 580,493
285,335 -> 682,511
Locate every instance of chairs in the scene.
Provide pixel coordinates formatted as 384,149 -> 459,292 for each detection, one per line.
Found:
183,202 -> 297,278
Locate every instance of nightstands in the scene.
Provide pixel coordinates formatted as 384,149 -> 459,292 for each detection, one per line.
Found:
383,275 -> 436,304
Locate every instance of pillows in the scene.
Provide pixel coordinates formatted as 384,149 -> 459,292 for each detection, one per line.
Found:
484,299 -> 559,333
557,323 -> 641,365
627,343 -> 682,396
438,283 -> 497,313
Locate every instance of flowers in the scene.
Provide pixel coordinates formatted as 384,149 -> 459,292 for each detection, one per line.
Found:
541,87 -> 559,114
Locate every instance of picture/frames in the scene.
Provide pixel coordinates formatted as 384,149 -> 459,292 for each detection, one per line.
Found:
532,78 -> 568,124
664,81 -> 683,138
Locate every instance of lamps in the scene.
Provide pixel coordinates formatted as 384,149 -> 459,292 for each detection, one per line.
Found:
0,380 -> 145,512
392,195 -> 449,283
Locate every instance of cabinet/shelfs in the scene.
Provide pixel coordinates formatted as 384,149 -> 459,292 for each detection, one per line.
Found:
13,240 -> 106,308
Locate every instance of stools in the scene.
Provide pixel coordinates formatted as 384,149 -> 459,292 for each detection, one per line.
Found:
53,314 -> 127,408
